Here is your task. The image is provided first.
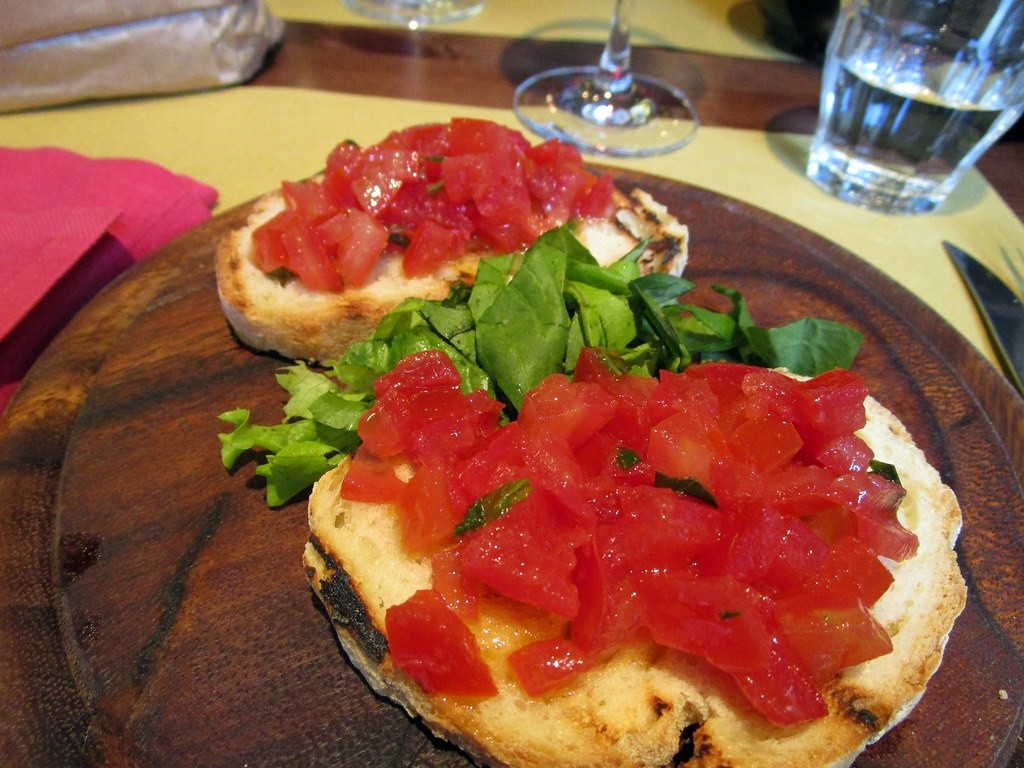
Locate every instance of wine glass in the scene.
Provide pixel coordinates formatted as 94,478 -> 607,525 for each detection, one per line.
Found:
510,1 -> 700,157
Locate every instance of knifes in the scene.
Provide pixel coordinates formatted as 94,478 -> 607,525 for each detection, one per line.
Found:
942,240 -> 1024,391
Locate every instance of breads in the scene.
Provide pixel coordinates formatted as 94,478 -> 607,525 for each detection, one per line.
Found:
215,174 -> 690,363
300,368 -> 967,767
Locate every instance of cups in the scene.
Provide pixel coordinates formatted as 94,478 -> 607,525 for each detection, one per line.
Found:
806,1 -> 1023,211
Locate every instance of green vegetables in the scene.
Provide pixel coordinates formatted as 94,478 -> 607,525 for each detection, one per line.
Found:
217,224 -> 863,507
